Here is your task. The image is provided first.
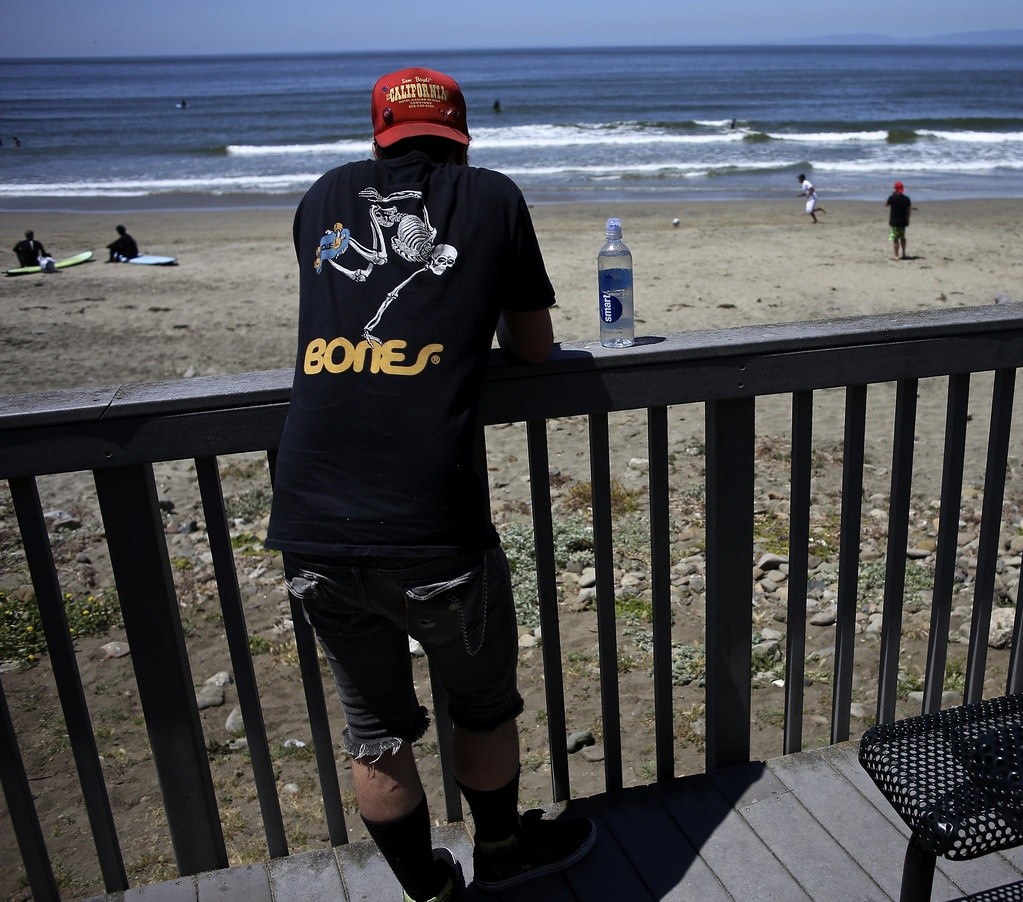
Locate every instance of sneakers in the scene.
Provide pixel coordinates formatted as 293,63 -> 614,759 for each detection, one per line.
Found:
473,808 -> 597,891
400,846 -> 456,902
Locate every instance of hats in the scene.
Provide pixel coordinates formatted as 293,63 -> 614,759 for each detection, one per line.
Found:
370,67 -> 469,148
894,182 -> 904,194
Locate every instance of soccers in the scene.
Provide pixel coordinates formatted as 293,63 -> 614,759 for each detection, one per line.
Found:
673,218 -> 680,226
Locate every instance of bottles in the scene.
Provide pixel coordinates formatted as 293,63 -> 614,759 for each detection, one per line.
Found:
598,218 -> 634,348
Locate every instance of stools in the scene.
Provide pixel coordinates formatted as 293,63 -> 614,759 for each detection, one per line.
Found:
859,689 -> 1023,902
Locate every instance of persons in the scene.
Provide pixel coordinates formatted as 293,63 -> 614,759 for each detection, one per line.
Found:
797,174 -> 827,223
264,66 -> 597,902
13,231 -> 51,267
731,117 -> 736,128
14,136 -> 20,145
182,100 -> 186,105
493,99 -> 500,109
105,225 -> 138,263
885,182 -> 911,259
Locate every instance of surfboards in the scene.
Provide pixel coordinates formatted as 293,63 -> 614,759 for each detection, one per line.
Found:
115,253 -> 176,265
1,250 -> 93,274
